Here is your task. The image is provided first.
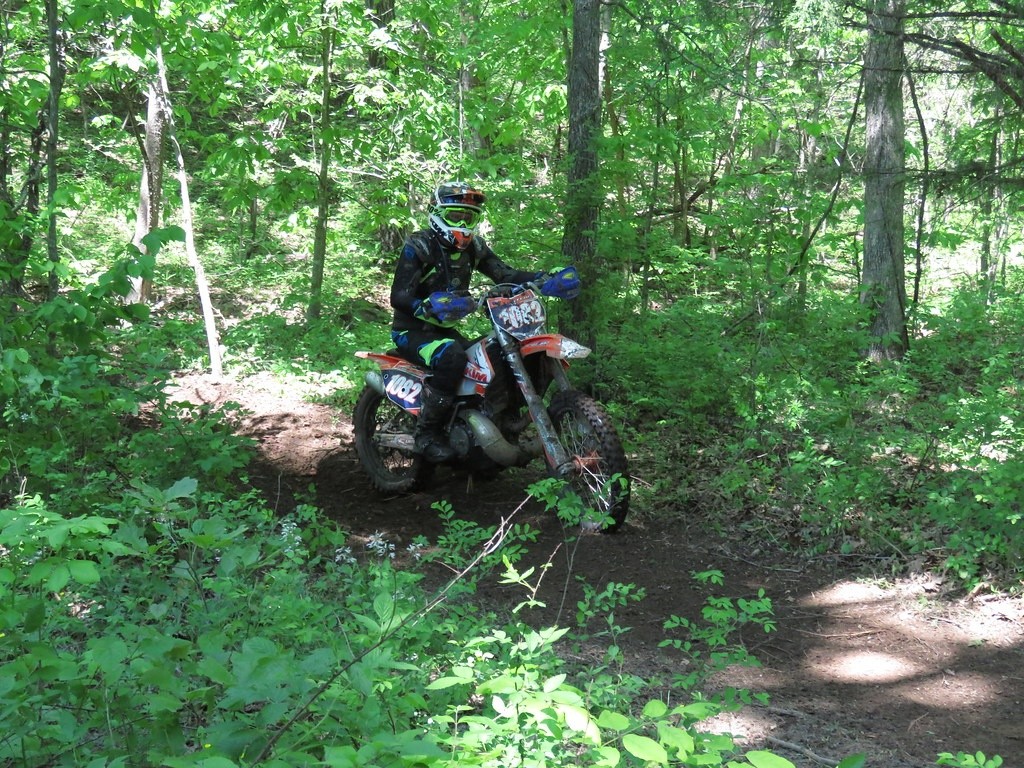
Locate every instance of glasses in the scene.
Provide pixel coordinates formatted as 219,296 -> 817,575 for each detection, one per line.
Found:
443,207 -> 481,229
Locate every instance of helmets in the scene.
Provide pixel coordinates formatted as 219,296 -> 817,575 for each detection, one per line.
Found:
428,181 -> 486,252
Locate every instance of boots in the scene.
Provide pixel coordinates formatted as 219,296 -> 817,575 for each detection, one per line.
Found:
413,375 -> 458,460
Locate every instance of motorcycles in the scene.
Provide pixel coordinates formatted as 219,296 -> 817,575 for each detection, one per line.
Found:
353,266 -> 633,537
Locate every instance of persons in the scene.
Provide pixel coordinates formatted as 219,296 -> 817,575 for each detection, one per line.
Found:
389,183 -> 577,466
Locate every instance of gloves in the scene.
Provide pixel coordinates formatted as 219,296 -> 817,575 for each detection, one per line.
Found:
548,266 -> 565,275
422,298 -> 435,319
